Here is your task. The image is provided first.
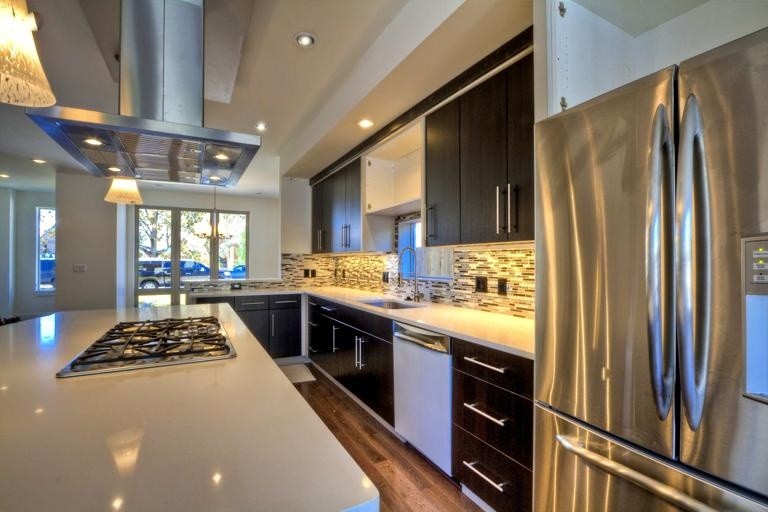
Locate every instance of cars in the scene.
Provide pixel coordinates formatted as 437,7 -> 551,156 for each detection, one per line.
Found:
228,264 -> 247,279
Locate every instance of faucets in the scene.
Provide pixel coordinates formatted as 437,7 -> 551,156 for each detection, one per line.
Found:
398,247 -> 424,303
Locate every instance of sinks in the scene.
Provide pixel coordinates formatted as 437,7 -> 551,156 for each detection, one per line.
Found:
357,297 -> 429,310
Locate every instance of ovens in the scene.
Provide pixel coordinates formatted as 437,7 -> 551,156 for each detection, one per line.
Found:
391,318 -> 453,480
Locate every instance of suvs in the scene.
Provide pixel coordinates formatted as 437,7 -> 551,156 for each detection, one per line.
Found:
40,258 -> 56,288
137,258 -> 233,290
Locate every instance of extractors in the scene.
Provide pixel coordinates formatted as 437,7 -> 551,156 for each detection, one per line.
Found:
24,0 -> 264,190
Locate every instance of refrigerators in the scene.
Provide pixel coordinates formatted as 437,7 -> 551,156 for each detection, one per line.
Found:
536,26 -> 768,510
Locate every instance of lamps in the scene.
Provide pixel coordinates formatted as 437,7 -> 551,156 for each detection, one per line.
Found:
0,1 -> 60,108
101,178 -> 146,207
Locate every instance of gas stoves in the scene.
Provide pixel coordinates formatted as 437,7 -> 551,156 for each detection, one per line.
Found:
54,316 -> 237,378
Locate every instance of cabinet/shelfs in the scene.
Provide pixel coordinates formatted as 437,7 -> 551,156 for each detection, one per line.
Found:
304,291 -> 343,391
329,154 -> 395,254
268,293 -> 303,360
446,327 -> 534,511
338,301 -> 395,435
308,172 -> 334,254
424,95 -> 460,250
196,295 -> 269,353
361,113 -> 429,218
458,48 -> 536,246
